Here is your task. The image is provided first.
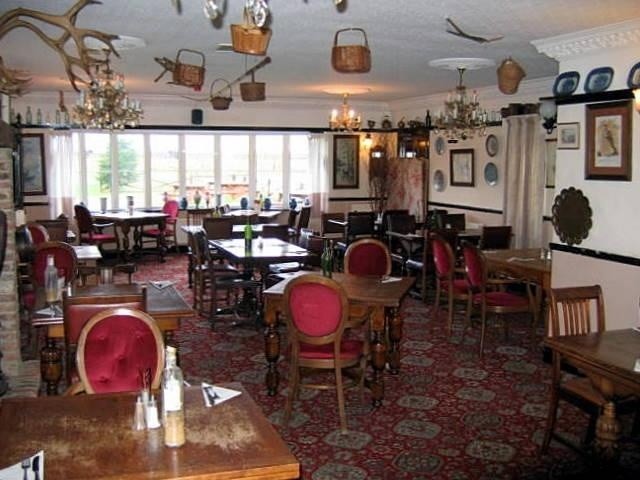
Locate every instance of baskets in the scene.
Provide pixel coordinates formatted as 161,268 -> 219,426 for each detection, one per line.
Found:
231,7 -> 273,55
496,57 -> 528,93
332,27 -> 372,73
173,48 -> 205,89
210,78 -> 233,111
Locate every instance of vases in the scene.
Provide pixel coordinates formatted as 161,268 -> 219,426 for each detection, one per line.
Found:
80,189 -> 310,215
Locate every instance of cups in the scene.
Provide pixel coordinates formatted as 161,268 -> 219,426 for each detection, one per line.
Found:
480,110 -> 501,123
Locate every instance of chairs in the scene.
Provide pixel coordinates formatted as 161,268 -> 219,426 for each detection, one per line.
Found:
17,204 -> 551,434
541,285 -> 639,464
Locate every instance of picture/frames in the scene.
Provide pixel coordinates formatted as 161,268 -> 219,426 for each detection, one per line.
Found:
13,134 -> 46,195
332,135 -> 358,187
558,123 -> 580,148
449,149 -> 473,186
584,99 -> 632,181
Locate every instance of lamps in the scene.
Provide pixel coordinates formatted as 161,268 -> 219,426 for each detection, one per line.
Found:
324,89 -> 362,133
71,35 -> 145,131
428,58 -> 502,140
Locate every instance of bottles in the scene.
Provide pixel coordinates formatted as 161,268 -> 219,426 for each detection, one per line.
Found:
320,239 -> 332,278
259,194 -> 264,210
424,109 -> 432,127
540,247 -> 551,260
126,195 -> 135,218
44,253 -> 59,302
212,207 -> 222,218
244,220 -> 253,249
130,397 -> 146,431
431,208 -> 438,229
66,280 -> 74,298
146,393 -> 162,430
24,106 -> 70,125
161,346 -> 185,412
163,412 -> 186,449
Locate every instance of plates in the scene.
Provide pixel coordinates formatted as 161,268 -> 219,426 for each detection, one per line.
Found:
626,61 -> 640,89
552,71 -> 580,96
432,169 -> 446,192
484,161 -> 501,186
485,134 -> 498,157
436,136 -> 443,155
583,67 -> 615,93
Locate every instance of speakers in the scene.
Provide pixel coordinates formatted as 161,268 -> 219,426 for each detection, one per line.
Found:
192,110 -> 202,124
